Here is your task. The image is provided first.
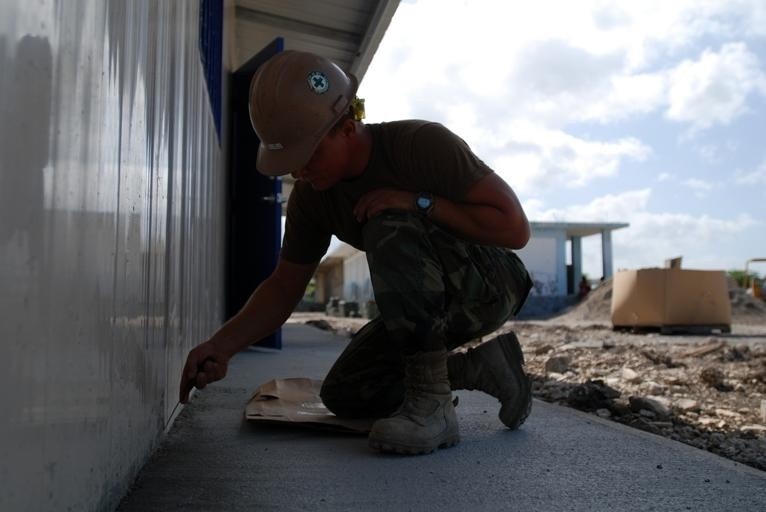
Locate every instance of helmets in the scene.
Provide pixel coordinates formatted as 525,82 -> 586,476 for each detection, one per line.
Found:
247,50 -> 358,177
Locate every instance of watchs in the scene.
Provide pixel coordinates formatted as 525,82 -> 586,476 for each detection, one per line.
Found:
410,189 -> 438,221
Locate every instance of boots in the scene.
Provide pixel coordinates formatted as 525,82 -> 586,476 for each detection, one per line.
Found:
448,331 -> 532,431
367,349 -> 459,456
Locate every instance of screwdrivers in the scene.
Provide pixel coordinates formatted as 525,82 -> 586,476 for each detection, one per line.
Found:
163,356 -> 215,430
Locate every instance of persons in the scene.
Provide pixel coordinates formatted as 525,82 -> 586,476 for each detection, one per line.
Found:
176,48 -> 531,455
